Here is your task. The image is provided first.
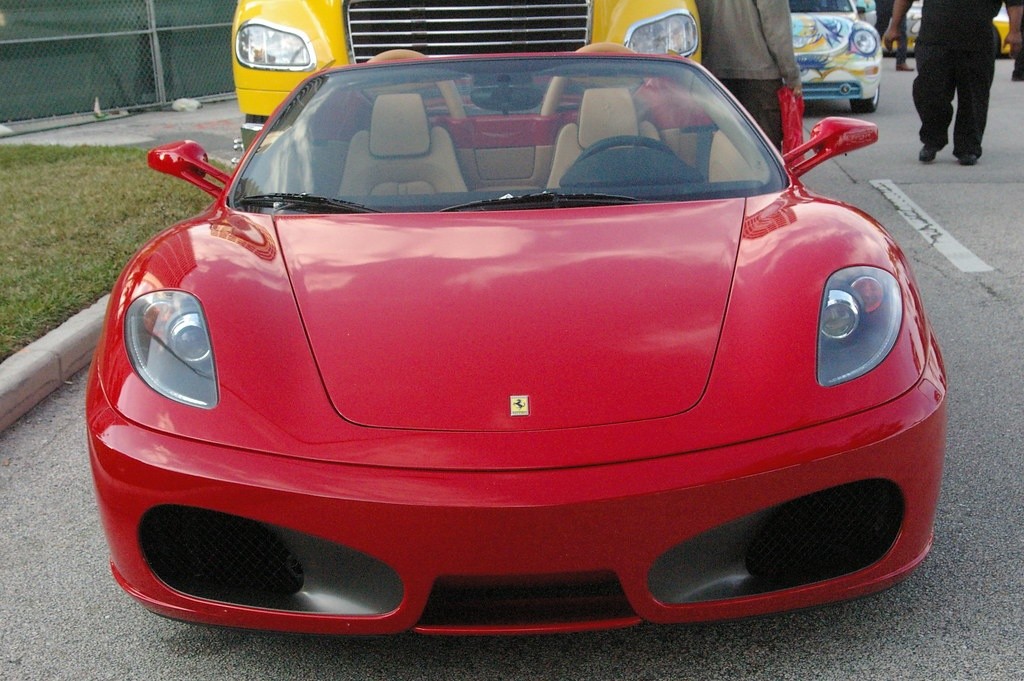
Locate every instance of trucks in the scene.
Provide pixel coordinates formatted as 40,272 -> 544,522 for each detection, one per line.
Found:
230,0 -> 702,158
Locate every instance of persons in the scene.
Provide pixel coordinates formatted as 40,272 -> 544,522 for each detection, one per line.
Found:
695,1 -> 802,153
873,0 -> 915,72
883,0 -> 1024,166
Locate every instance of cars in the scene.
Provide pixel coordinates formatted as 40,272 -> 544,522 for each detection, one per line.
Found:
787,1 -> 885,110
879,0 -> 1012,59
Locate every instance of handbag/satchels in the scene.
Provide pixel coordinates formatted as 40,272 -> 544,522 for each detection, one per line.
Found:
776,86 -> 806,170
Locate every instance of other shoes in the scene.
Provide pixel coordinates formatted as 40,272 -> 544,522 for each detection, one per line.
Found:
895,63 -> 914,71
1012,71 -> 1024,81
919,144 -> 940,162
958,152 -> 977,165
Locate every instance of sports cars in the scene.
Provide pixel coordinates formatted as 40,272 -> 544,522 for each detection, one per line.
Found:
85,41 -> 949,637
